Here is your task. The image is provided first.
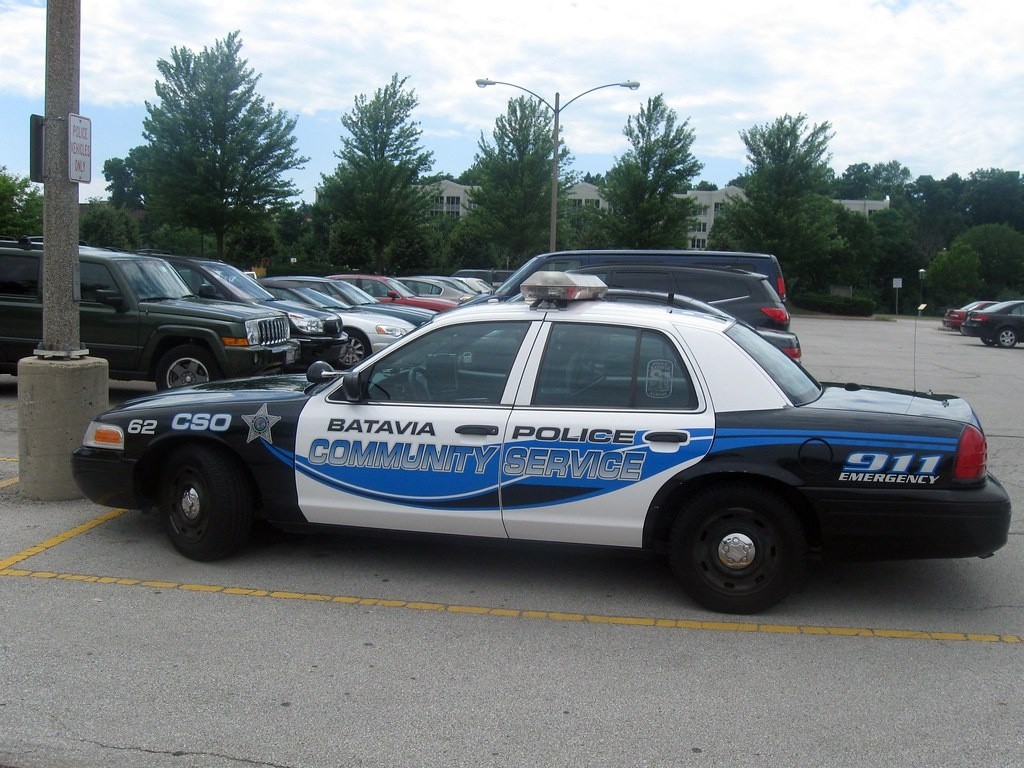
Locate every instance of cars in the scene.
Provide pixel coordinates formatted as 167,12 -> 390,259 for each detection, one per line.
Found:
325,275 -> 459,313
504,289 -> 802,365
72,276 -> 1012,614
105,247 -> 349,368
257,280 -> 418,370
564,266 -> 802,331
942,301 -> 1000,336
392,276 -> 495,304
961,300 -> 1024,349
257,276 -> 440,328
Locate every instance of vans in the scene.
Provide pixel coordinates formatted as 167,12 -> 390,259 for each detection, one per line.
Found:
457,250 -> 786,309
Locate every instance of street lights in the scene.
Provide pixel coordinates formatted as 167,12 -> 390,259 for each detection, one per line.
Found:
476,78 -> 640,271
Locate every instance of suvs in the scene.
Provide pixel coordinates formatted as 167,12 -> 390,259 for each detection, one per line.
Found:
0,236 -> 301,392
